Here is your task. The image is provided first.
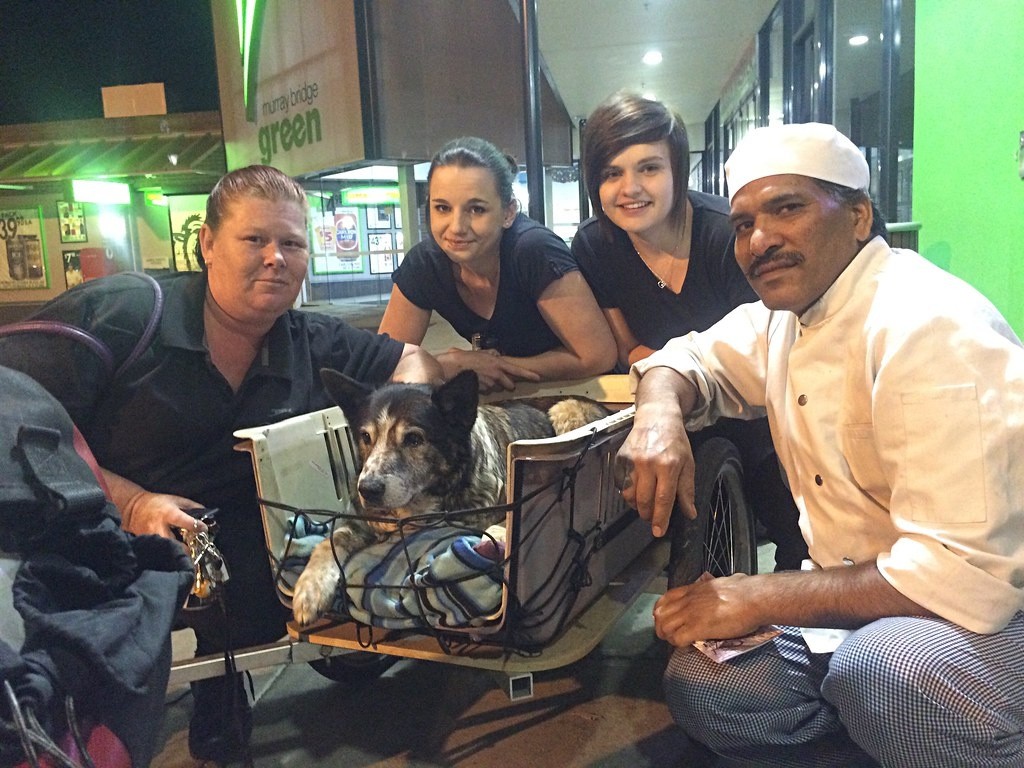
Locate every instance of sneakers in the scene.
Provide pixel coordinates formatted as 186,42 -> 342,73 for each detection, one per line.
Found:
190,670 -> 256,758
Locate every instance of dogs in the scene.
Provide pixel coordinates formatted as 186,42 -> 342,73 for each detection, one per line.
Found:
291,367 -> 620,628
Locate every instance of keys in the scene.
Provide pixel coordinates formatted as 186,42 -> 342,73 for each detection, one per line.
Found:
183,507 -> 223,593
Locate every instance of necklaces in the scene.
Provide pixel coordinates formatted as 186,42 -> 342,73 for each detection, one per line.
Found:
629,203 -> 688,289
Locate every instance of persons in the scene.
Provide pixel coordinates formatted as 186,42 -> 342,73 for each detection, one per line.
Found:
571,85 -> 811,573
0,166 -> 444,768
614,115 -> 1024,768
378,135 -> 617,395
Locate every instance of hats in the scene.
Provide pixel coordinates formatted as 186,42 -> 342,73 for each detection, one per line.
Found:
722,121 -> 875,207
